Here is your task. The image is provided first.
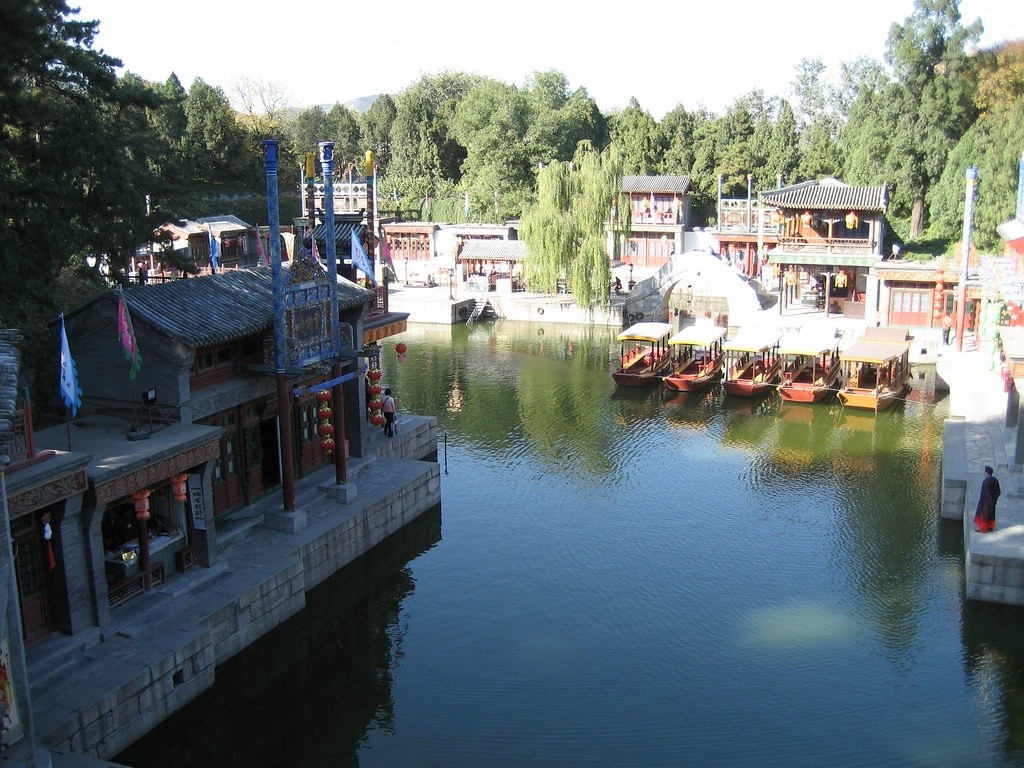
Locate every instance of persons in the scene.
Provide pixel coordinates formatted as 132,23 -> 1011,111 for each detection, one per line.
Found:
381,388 -> 397,438
973,466 -> 1001,533
941,312 -> 952,347
613,277 -> 622,296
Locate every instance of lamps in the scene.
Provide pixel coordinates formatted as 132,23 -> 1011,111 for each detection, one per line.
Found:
142,390 -> 156,404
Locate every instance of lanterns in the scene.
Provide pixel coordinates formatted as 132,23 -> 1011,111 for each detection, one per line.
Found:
133,490 -> 150,519
316,389 -> 335,457
367,367 -> 386,429
396,342 -> 407,362
171,474 -> 187,502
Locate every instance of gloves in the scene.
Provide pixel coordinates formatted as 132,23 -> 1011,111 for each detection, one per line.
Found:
993,496 -> 997,505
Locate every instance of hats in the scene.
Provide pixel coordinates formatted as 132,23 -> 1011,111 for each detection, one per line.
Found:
985,465 -> 993,473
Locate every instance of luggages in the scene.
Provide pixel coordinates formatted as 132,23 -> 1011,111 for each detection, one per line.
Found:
391,412 -> 400,437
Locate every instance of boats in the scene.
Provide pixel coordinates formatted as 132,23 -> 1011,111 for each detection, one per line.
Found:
835,340 -> 915,412
662,325 -> 727,393
775,335 -> 841,403
610,322 -> 673,386
721,331 -> 783,397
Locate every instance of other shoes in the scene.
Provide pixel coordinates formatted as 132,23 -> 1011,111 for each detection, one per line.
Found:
975,528 -> 993,533
384,428 -> 387,435
388,431 -> 393,437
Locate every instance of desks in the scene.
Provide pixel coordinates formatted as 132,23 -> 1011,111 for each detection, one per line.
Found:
105,530 -> 185,578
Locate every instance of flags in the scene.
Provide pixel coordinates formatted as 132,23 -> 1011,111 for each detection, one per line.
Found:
118,292 -> 142,379
204,226 -> 396,286
61,325 -> 83,418
393,186 -> 499,217
